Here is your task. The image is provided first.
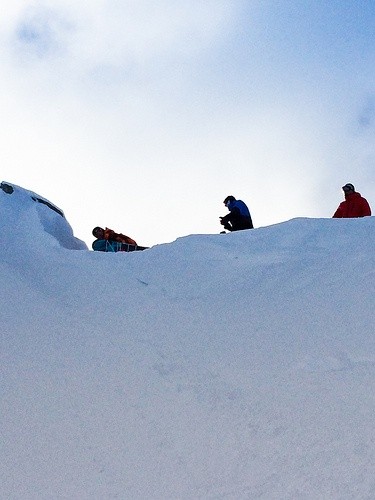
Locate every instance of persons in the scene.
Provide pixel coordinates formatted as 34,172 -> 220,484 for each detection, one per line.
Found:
92,227 -> 137,245
332,183 -> 371,218
219,196 -> 254,233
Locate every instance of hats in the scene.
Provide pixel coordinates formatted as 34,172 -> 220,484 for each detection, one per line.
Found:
342,183 -> 355,192
